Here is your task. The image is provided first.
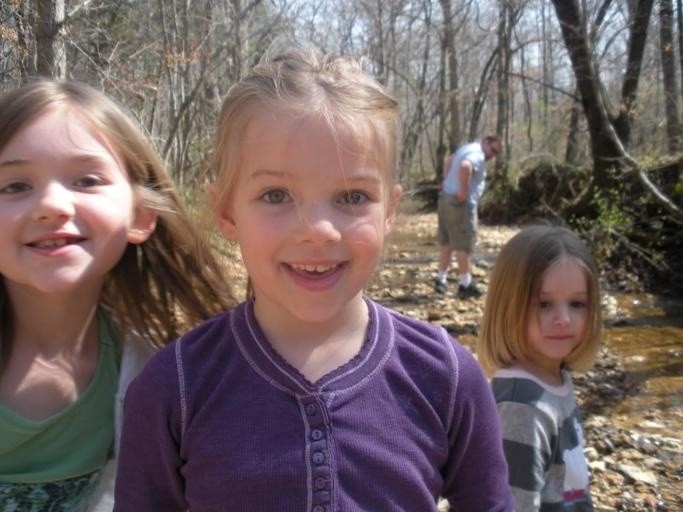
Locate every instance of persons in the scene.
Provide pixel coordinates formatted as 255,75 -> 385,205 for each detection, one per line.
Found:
112,30 -> 515,512
0,72 -> 241,511
474,226 -> 611,510
424,132 -> 499,301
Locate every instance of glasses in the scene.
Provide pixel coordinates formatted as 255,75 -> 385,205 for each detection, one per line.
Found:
489,144 -> 498,155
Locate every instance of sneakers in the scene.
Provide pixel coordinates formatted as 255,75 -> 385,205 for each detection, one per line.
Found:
457,281 -> 484,300
434,278 -> 448,295
